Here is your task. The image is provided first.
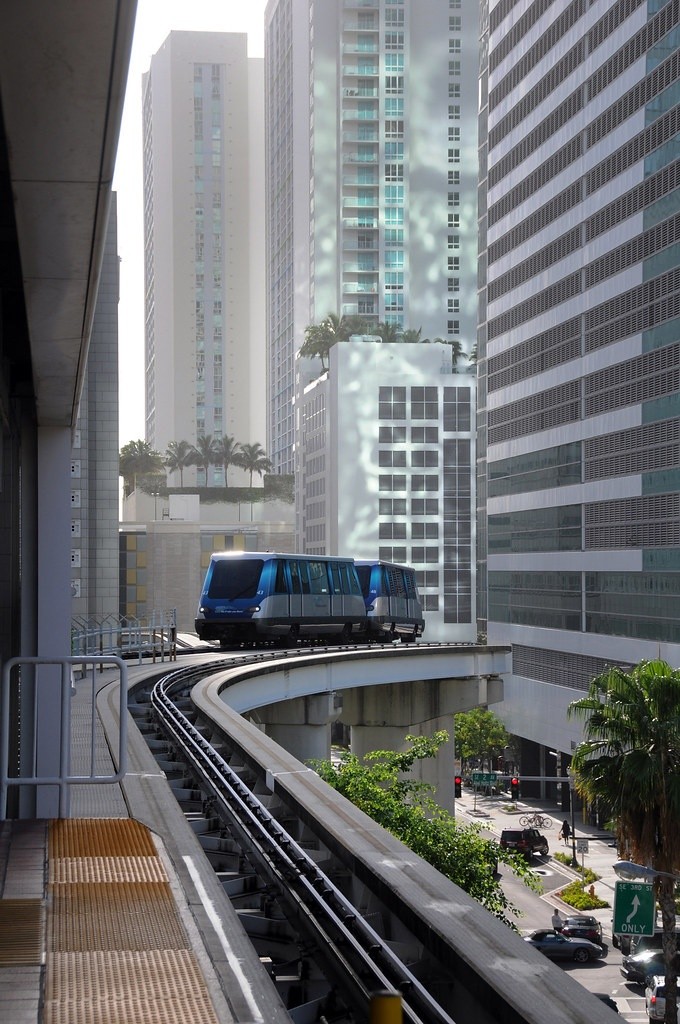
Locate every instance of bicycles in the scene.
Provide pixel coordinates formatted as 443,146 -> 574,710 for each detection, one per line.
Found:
519,811 -> 552,828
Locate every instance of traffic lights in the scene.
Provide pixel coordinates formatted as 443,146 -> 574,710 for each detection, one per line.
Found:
455,776 -> 461,798
511,778 -> 519,799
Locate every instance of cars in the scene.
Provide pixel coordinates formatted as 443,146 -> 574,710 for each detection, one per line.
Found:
524,929 -> 603,962
562,916 -> 602,945
620,949 -> 680,987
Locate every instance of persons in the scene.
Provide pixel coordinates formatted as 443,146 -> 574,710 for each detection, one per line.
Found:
559,820 -> 570,846
552,909 -> 565,933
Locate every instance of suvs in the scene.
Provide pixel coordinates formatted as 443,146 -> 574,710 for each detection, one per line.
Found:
500,827 -> 549,859
645,975 -> 680,1021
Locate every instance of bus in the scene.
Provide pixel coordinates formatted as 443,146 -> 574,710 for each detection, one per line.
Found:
195,552 -> 425,647
